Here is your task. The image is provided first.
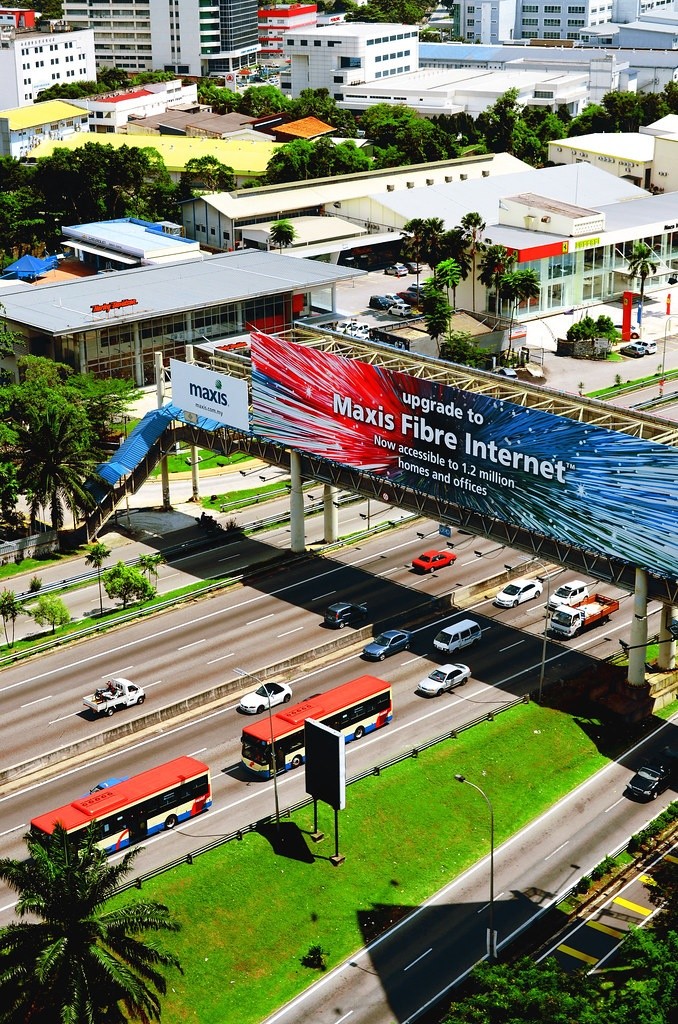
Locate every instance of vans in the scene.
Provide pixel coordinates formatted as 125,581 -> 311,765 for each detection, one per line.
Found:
548,580 -> 590,612
433,618 -> 482,656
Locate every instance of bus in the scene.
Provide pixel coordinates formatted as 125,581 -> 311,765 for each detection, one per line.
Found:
240,674 -> 394,779
29,756 -> 213,859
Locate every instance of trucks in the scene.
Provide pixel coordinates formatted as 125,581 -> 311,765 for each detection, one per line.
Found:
82,678 -> 146,717
549,593 -> 619,638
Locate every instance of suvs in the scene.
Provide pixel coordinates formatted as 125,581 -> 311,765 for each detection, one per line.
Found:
633,339 -> 657,355
324,601 -> 368,629
620,344 -> 645,358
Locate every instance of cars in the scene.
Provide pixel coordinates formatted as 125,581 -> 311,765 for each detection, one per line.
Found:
327,280 -> 429,341
80,774 -> 131,799
362,628 -> 416,662
239,682 -> 292,714
498,367 -> 519,380
405,261 -> 423,274
417,663 -> 472,697
412,549 -> 458,573
624,756 -> 677,802
385,265 -> 408,276
495,578 -> 543,608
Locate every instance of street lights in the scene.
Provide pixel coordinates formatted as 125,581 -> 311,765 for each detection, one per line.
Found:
517,556 -> 550,704
454,773 -> 494,965
232,667 -> 280,833
